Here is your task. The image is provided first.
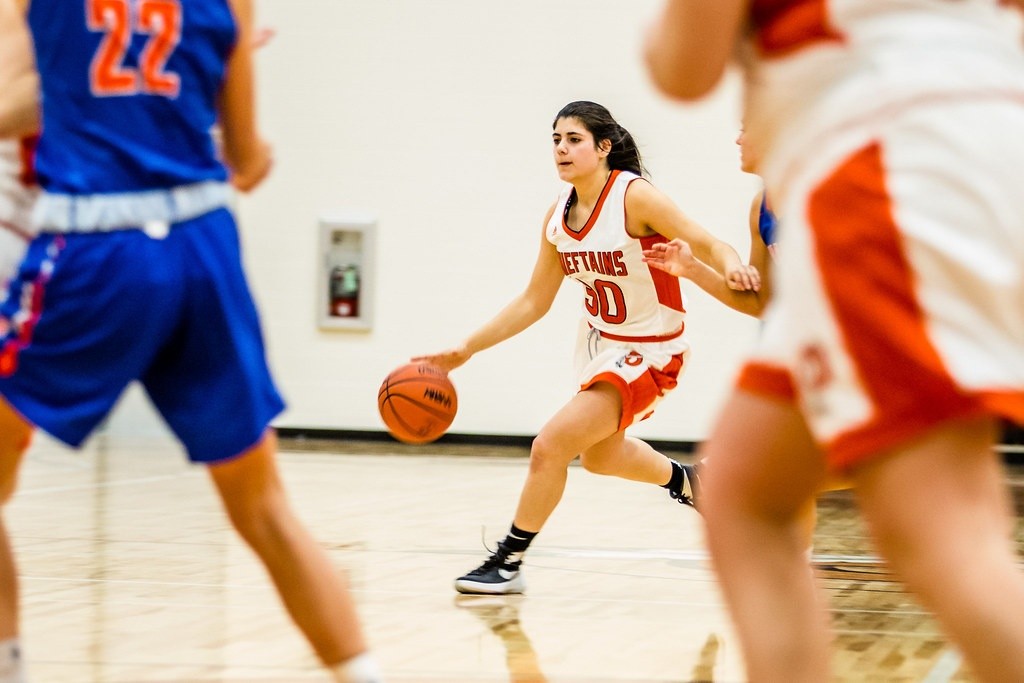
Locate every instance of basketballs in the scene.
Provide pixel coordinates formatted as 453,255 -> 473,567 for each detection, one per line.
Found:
376,360 -> 459,447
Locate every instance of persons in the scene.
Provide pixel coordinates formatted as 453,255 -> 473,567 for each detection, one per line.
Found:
1,0 -> 378,683
411,101 -> 761,594
457,591 -> 547,683
639,116 -> 786,318
642,1 -> 1024,683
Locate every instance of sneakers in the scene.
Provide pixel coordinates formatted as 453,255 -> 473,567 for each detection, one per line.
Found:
455,526 -> 524,593
670,457 -> 710,516
